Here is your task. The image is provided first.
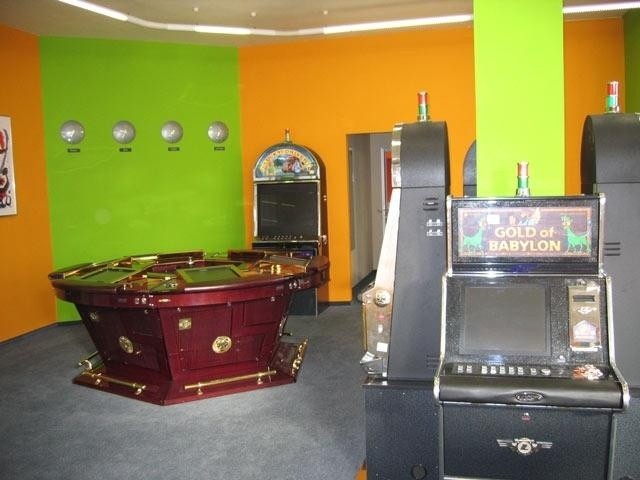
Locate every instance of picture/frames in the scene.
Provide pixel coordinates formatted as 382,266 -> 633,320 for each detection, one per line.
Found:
0,116 -> 18,216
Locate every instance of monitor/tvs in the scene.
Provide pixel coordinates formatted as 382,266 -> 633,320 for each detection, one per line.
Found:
253,179 -> 321,240
444,275 -> 571,368
